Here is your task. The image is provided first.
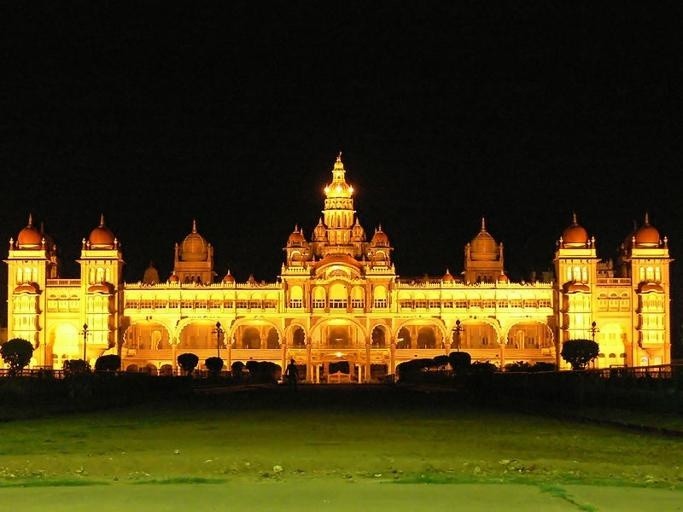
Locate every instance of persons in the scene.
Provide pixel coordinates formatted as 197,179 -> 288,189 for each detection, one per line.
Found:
283,359 -> 299,397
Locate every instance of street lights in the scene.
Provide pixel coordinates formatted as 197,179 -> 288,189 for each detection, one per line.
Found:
588,321 -> 599,369
77,323 -> 91,362
452,319 -> 465,351
211,321 -> 223,358
